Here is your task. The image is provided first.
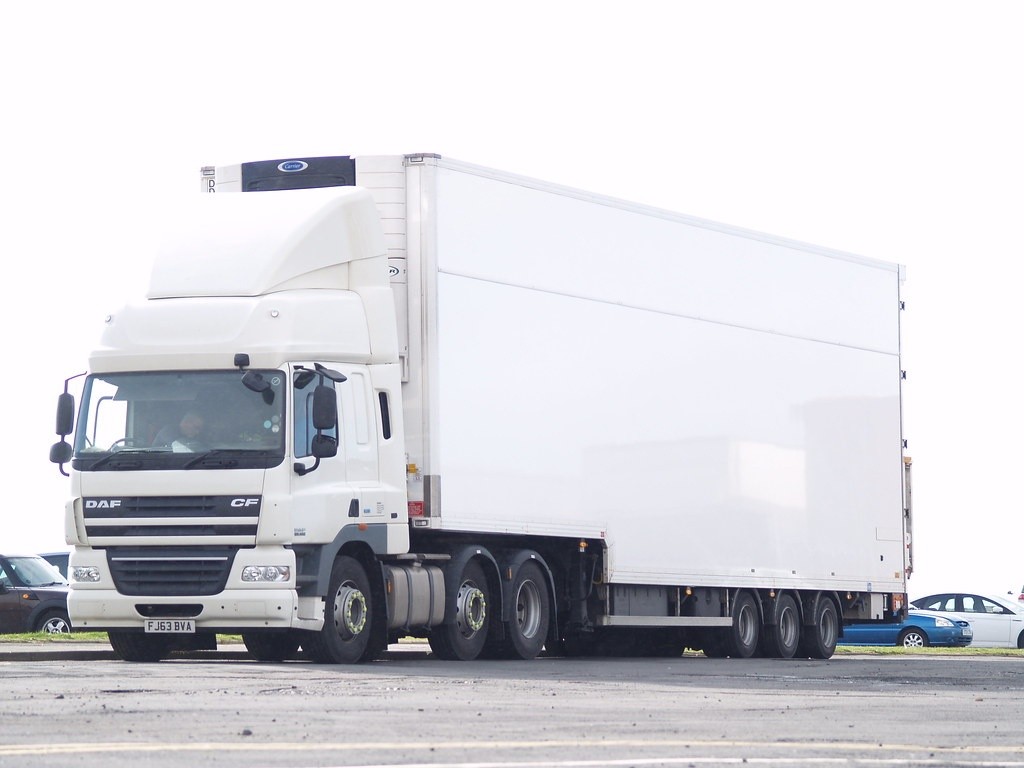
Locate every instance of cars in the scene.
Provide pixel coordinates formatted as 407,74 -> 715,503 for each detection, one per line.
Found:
836,603 -> 973,647
0,553 -> 83,635
908,591 -> 1024,649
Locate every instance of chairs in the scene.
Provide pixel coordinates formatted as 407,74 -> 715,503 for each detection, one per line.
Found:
138,408 -> 173,444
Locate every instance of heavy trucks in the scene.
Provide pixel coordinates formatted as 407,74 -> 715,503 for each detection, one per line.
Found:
50,153 -> 914,665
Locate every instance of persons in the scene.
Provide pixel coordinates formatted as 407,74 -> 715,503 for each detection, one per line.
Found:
151,412 -> 204,447
